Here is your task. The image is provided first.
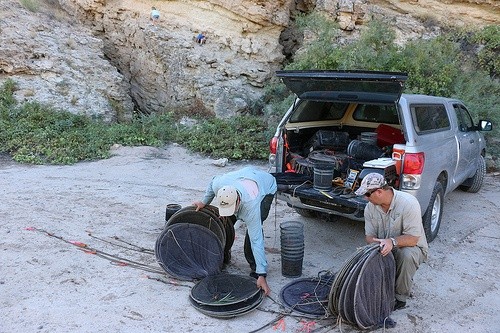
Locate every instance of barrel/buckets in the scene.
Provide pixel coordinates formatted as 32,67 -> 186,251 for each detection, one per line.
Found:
393,143 -> 406,187
313,168 -> 334,192
279,220 -> 305,278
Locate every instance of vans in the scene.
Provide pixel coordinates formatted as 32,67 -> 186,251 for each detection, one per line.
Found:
267,70 -> 493,243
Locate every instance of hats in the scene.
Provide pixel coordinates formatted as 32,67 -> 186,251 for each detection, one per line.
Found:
216,185 -> 238,217
354,172 -> 387,196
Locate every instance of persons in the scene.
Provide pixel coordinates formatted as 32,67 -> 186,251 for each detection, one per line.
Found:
192,166 -> 277,298
355,173 -> 428,310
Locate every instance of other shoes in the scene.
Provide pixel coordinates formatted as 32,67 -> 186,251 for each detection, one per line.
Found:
393,299 -> 406,310
249,272 -> 259,279
222,259 -> 231,269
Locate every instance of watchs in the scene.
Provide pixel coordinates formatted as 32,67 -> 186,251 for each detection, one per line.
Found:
391,238 -> 396,249
258,273 -> 267,278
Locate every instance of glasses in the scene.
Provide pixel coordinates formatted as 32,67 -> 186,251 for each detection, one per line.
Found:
364,188 -> 379,197
